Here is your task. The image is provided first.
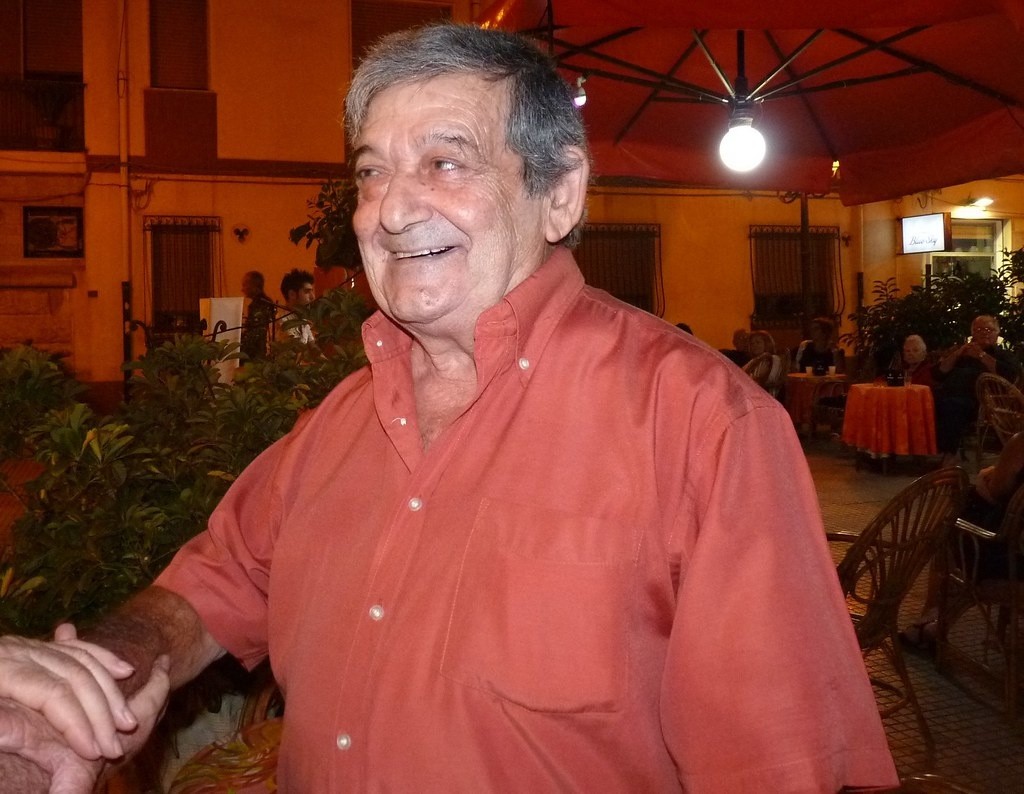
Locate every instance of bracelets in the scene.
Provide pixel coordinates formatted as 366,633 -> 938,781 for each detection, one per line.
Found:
979,352 -> 986,358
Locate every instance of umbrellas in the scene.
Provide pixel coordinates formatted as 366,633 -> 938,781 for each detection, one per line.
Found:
472,0 -> 1024,210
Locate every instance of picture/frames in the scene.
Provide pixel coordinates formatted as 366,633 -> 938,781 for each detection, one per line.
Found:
22,206 -> 83,257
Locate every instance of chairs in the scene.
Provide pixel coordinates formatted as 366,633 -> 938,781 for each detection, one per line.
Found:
130,304 -> 277,400
741,340 -> 1024,794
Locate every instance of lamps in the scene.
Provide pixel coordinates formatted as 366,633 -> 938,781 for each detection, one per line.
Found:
573,76 -> 586,106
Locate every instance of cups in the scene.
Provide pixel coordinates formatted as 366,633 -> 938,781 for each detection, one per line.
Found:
805,366 -> 813,376
828,366 -> 837,376
904,375 -> 912,386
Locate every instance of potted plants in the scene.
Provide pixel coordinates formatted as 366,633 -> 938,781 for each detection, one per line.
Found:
21,73 -> 87,148
0,289 -> 375,794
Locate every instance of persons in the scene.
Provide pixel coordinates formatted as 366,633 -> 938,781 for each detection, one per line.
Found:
0,27 -> 904,794
0,620 -> 172,794
241,271 -> 277,359
268,267 -> 315,344
897,334 -> 935,387
931,315 -> 1018,467
790,317 -> 845,376
898,431 -> 1024,661
675,323 -> 783,398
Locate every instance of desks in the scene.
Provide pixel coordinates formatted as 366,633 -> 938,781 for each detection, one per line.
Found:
843,382 -> 937,474
784,373 -> 848,445
